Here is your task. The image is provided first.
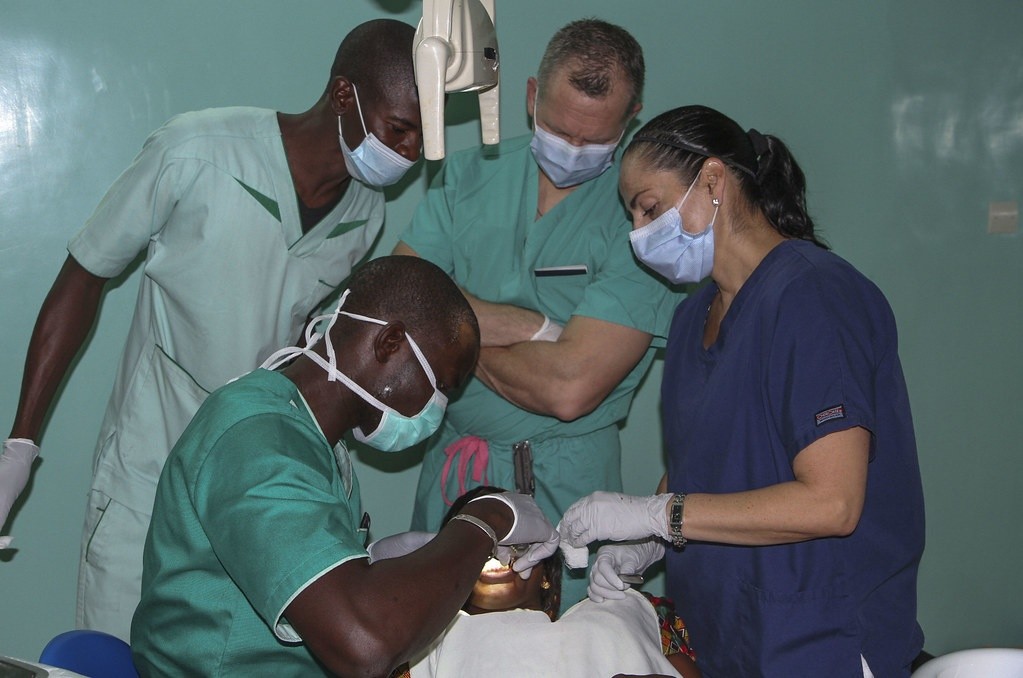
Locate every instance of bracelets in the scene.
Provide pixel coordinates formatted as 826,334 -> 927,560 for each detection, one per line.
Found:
448,512 -> 500,563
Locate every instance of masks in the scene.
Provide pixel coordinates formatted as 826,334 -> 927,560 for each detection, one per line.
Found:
528,85 -> 631,189
337,81 -> 419,188
222,289 -> 449,453
629,168 -> 719,286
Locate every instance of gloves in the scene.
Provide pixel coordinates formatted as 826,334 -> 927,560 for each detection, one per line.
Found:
468,491 -> 561,580
559,489 -> 674,543
366,529 -> 438,566
0,437 -> 42,550
587,533 -> 666,603
530,313 -> 563,342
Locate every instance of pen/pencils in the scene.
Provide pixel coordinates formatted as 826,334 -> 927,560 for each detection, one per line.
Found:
361,512 -> 371,542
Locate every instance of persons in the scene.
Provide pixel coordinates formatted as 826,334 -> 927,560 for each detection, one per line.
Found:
1,17 -> 450,645
561,105 -> 927,678
129,255 -> 562,678
385,485 -> 703,678
386,17 -> 689,620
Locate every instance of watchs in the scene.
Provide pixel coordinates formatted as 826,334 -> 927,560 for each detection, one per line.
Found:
668,491 -> 689,549
